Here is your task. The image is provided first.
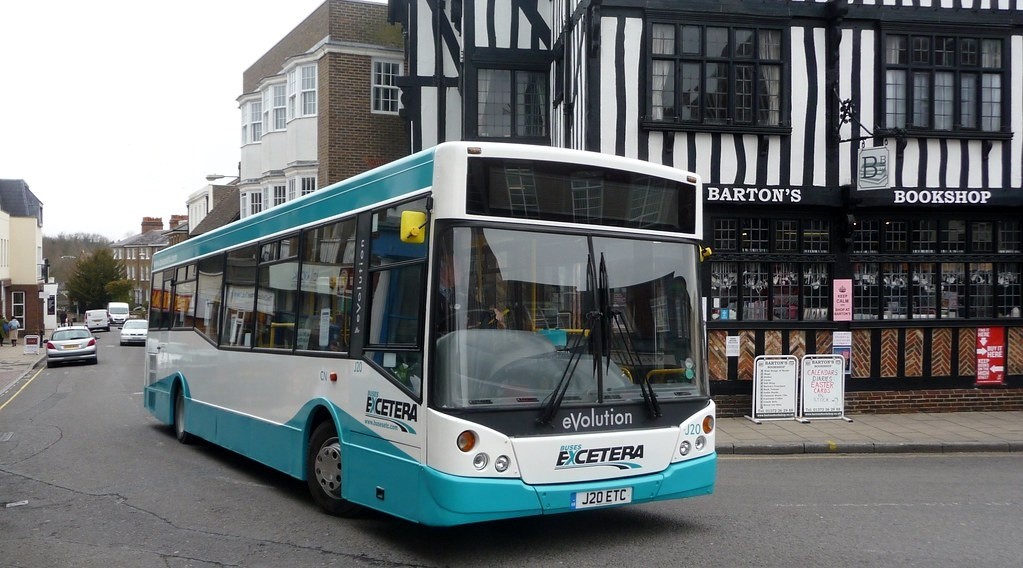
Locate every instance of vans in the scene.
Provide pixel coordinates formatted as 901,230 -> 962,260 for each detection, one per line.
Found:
84,310 -> 112,333
107,302 -> 130,327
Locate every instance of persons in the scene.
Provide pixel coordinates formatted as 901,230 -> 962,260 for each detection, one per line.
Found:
436,255 -> 505,337
60,312 -> 67,327
67,311 -> 73,327
0,315 -> 9,346
8,316 -> 20,347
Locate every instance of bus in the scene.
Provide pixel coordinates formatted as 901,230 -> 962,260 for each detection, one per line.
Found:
142,141 -> 718,527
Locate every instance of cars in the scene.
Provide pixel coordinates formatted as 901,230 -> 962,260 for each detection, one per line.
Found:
118,318 -> 148,346
43,325 -> 100,368
